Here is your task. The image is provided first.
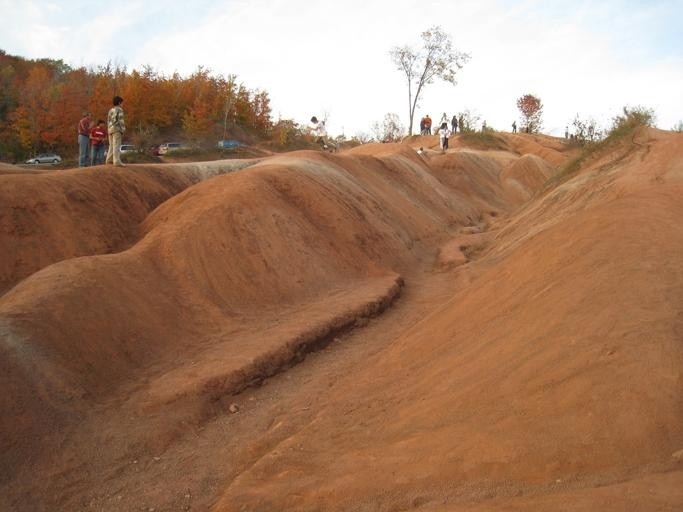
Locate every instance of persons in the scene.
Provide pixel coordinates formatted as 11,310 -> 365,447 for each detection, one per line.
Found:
416,110 -> 465,154
89,119 -> 107,165
305,114 -> 338,151
510,121 -> 517,134
480,119 -> 486,131
104,95 -> 130,167
76,111 -> 91,166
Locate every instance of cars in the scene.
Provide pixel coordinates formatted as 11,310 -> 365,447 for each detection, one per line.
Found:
119,144 -> 136,154
218,140 -> 238,148
148,143 -> 187,155
26,154 -> 61,164
